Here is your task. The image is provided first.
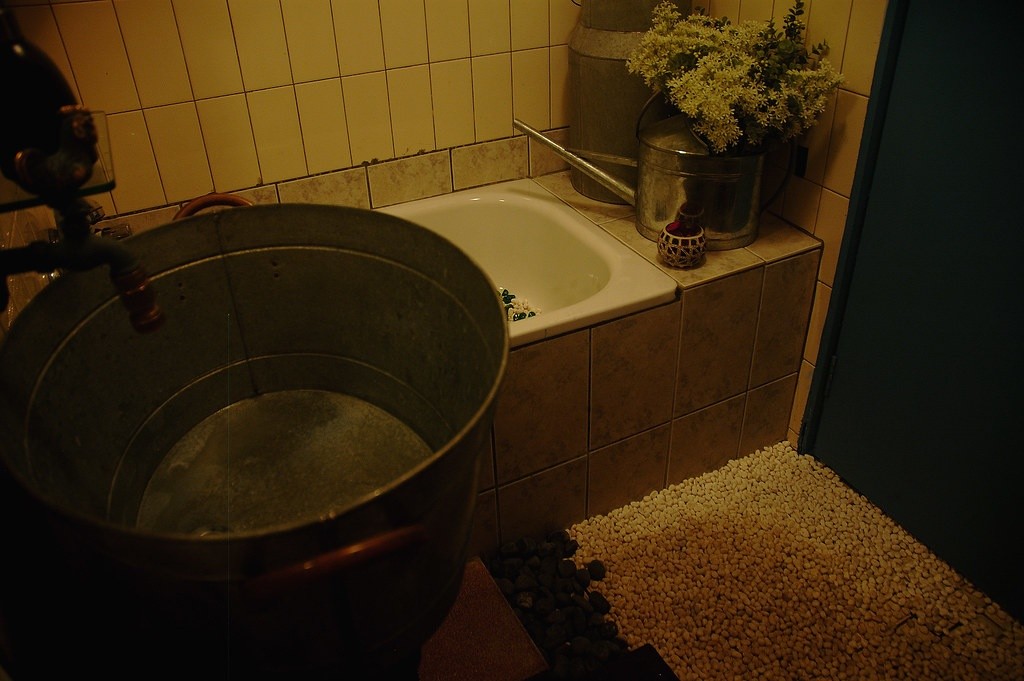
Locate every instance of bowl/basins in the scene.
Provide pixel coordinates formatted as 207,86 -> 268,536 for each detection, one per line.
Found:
0,196 -> 510,681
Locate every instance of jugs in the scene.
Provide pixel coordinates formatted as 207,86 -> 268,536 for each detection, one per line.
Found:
512,83 -> 765,251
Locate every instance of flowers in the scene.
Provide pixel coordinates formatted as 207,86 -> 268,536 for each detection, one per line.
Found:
621,0 -> 843,155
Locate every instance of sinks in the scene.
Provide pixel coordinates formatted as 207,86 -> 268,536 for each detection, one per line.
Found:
369,175 -> 680,352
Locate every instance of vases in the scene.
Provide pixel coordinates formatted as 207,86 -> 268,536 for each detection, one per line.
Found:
634,90 -> 765,251
